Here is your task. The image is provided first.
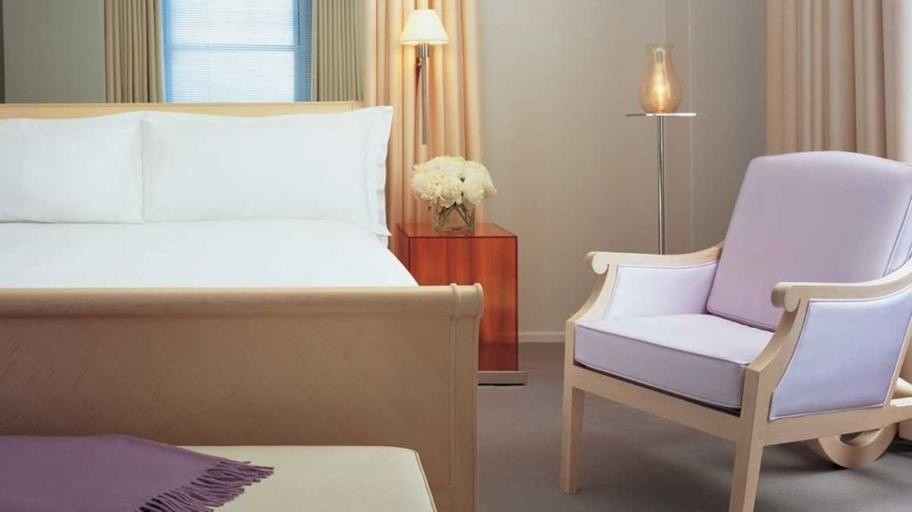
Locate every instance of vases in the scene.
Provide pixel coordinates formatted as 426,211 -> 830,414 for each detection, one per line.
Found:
430,202 -> 476,236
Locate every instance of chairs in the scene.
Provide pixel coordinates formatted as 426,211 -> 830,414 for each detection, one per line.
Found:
560,150 -> 911,512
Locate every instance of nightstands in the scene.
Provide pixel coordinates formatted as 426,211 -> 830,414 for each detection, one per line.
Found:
393,220 -> 528,387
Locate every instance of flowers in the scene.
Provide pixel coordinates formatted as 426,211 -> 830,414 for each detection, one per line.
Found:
409,153 -> 497,226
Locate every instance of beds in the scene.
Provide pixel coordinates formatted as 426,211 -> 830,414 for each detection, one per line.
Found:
0,101 -> 483,512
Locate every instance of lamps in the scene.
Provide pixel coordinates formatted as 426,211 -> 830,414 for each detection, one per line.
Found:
639,40 -> 683,113
400,10 -> 451,160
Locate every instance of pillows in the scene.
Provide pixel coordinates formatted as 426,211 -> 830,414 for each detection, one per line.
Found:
142,110 -> 395,236
4,106 -> 142,223
